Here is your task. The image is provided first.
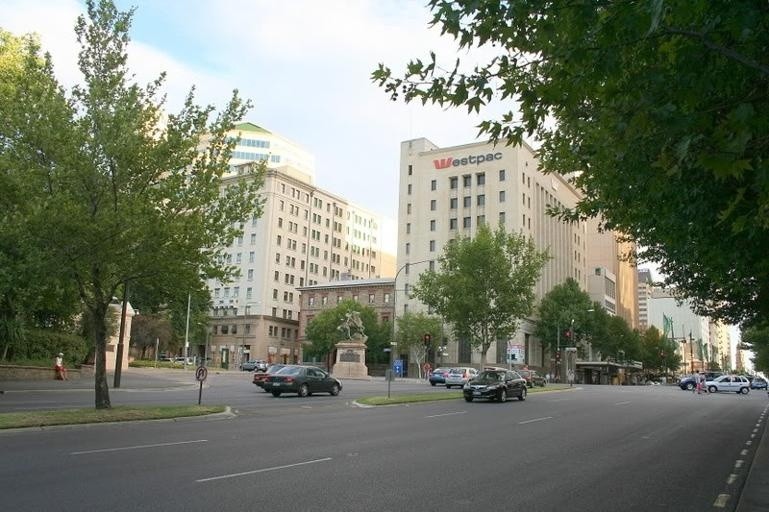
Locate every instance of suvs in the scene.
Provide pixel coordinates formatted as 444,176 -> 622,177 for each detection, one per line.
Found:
240,359 -> 268,372
680,370 -> 750,393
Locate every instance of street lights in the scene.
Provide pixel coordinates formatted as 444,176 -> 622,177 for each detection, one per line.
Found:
393,257 -> 442,360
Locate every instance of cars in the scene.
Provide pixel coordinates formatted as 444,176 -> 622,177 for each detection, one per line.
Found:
253,362 -> 343,398
430,365 -> 546,402
750,376 -> 767,390
159,351 -> 212,365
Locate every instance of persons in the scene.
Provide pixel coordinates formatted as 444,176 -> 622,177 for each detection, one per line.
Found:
692,369 -> 710,395
522,365 -> 529,370
423,360 -> 431,383
55,352 -> 70,382
